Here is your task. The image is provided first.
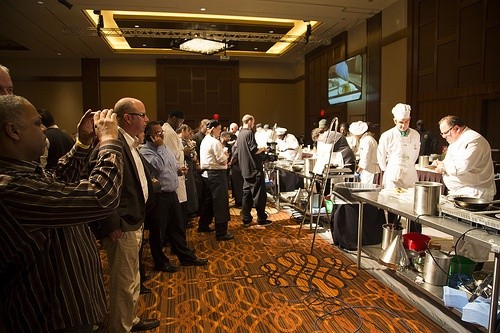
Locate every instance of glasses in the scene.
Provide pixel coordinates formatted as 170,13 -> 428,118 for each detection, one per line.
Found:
128,113 -> 147,119
153,132 -> 164,135
440,125 -> 456,136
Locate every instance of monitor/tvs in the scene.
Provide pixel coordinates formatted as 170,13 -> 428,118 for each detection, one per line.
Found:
326,53 -> 364,104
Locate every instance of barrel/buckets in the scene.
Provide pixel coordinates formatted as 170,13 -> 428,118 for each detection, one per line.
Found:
423,249 -> 453,286
419,156 -> 429,167
381,224 -> 403,250
312,193 -> 321,208
414,181 -> 443,215
404,233 -> 431,252
304,158 -> 317,173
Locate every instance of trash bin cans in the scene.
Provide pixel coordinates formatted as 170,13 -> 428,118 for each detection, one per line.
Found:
331,181 -> 383,250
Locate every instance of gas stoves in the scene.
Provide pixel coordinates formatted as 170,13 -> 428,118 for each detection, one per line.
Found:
437,203 -> 500,230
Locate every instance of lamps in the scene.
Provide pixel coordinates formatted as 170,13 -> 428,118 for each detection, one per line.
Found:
171,24 -> 238,55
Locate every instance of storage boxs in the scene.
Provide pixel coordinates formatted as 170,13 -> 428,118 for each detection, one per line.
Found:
448,255 -> 478,280
401,232 -> 431,250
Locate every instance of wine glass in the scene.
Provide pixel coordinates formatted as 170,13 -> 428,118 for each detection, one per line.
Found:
178,160 -> 187,180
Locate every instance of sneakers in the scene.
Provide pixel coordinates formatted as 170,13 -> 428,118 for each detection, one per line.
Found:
244,216 -> 252,226
257,220 -> 272,226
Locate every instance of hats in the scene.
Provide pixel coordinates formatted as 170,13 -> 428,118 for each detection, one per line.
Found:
349,121 -> 368,135
319,119 -> 328,128
392,103 -> 411,119
169,111 -> 184,119
275,128 -> 287,135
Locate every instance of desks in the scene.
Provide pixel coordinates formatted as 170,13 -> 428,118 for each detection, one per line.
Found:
274,163 -> 357,231
352,191 -> 500,333
416,164 -> 445,193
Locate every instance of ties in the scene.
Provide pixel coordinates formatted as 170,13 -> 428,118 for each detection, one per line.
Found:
401,132 -> 406,137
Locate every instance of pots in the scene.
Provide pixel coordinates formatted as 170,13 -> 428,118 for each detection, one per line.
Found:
453,196 -> 500,210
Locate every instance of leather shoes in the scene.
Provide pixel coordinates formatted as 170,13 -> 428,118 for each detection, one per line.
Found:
131,319 -> 160,331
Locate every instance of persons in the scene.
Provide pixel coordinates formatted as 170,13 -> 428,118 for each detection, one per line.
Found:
86,97 -> 160,333
313,119 -> 330,153
237,114 -> 273,227
37,108 -> 75,171
348,121 -> 381,184
139,121 -> 208,273
377,103 -> 422,233
275,128 -> 299,160
0,65 -> 13,96
192,119 -> 281,189
0,95 -> 124,333
197,121 -> 234,241
430,116 -> 497,206
311,128 -> 356,196
132,110 -> 196,255
340,123 -> 360,160
413,120 -> 438,156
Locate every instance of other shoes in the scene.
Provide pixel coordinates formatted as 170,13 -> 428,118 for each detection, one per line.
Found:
217,234 -> 234,241
184,224 -> 194,228
143,238 -> 148,245
158,266 -> 178,272
140,288 -> 151,294
182,259 -> 208,266
197,228 -> 215,232
143,275 -> 150,281
